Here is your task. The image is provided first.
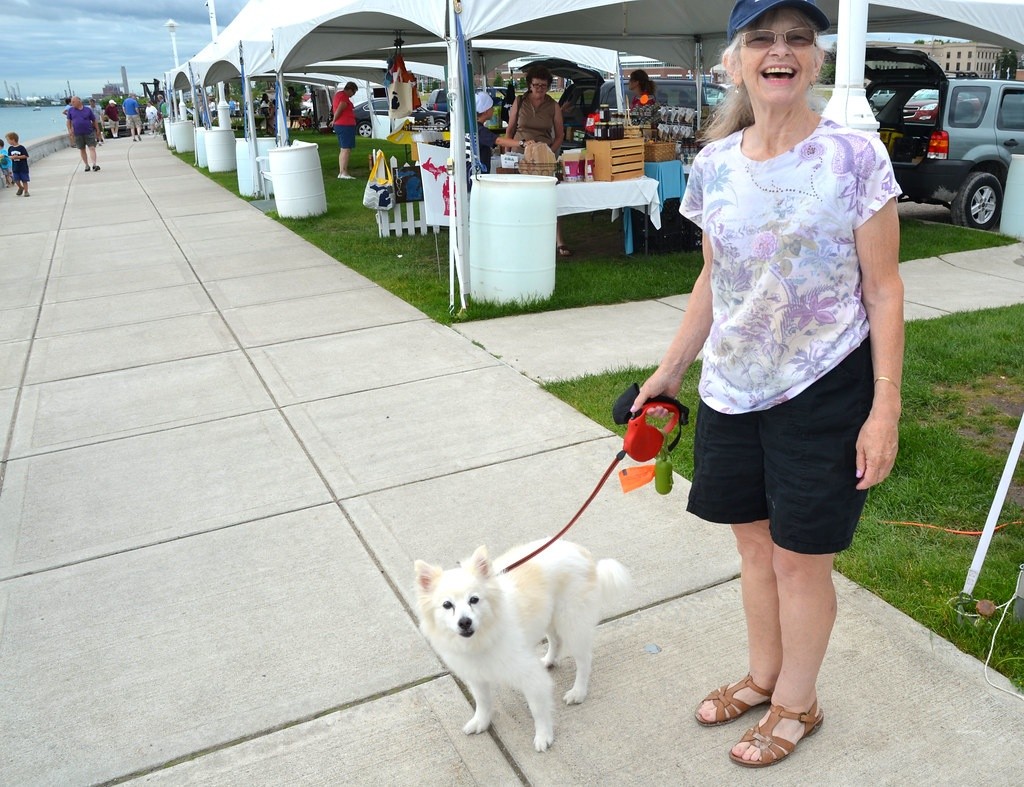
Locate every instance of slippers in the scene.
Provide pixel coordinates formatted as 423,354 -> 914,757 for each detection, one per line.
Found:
558,246 -> 570,256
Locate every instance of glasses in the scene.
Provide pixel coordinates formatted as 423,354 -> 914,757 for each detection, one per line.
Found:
531,82 -> 548,89
742,27 -> 817,49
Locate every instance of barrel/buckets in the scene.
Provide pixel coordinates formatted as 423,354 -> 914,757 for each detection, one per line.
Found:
195,126 -> 219,169
372,114 -> 390,140
395,117 -> 415,130
205,129 -> 237,173
268,143 -> 327,220
236,137 -> 277,200
468,173 -> 558,306
164,117 -> 194,154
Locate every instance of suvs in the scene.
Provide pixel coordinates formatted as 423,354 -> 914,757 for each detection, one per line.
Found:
864,46 -> 1024,231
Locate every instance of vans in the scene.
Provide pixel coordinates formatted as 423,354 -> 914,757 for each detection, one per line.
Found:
510,57 -> 734,143
426,86 -> 516,112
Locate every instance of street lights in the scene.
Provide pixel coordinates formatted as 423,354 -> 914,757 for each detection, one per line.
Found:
161,19 -> 187,121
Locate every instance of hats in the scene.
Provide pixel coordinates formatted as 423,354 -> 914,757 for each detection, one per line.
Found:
475,92 -> 493,113
728,0 -> 831,46
109,99 -> 116,105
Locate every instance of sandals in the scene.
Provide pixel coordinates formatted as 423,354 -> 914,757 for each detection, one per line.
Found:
694,673 -> 774,727
730,697 -> 824,769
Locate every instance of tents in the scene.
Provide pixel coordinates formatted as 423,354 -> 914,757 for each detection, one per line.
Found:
162,0 -> 1024,309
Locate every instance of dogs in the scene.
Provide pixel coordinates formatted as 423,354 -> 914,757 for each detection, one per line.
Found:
411,537 -> 634,752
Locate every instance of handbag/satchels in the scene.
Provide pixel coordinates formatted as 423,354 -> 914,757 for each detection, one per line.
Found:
363,151 -> 396,211
326,112 -> 334,126
393,56 -> 421,110
388,72 -> 413,119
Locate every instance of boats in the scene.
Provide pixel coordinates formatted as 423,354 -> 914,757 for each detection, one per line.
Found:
33,107 -> 41,111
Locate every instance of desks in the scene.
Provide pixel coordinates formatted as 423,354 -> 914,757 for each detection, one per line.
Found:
624,159 -> 687,253
557,175 -> 659,253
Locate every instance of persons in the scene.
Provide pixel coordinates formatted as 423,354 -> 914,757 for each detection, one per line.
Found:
62,98 -> 119,148
253,86 -> 301,133
145,94 -> 167,140
0,139 -> 15,188
122,93 -> 142,141
5,132 -> 30,196
67,96 -> 101,171
209,96 -> 241,117
476,66 -> 657,256
630,0 -> 904,765
329,81 -> 358,179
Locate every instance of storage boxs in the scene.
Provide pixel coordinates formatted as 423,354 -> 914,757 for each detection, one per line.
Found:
562,149 -> 594,180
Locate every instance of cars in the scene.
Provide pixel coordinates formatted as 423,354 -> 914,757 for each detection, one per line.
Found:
869,89 -> 981,124
301,98 -> 314,117
353,97 -> 450,138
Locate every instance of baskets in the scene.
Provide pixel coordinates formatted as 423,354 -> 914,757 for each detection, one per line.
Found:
644,142 -> 676,162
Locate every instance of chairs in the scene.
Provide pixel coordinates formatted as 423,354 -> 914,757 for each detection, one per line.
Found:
311,115 -> 323,132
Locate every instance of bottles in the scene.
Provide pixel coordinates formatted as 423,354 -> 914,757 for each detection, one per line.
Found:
403,118 -> 432,132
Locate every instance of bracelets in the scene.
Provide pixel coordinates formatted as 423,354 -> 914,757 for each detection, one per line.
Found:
874,377 -> 900,392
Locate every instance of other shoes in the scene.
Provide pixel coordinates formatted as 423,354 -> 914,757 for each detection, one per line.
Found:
148,132 -> 153,135
16,187 -> 24,195
25,192 -> 29,196
138,135 -> 141,141
133,139 -> 137,141
93,166 -> 100,171
341,175 -> 356,180
85,166 -> 90,171
337,174 -> 341,178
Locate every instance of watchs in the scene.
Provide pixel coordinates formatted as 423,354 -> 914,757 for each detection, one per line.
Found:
519,139 -> 526,148
